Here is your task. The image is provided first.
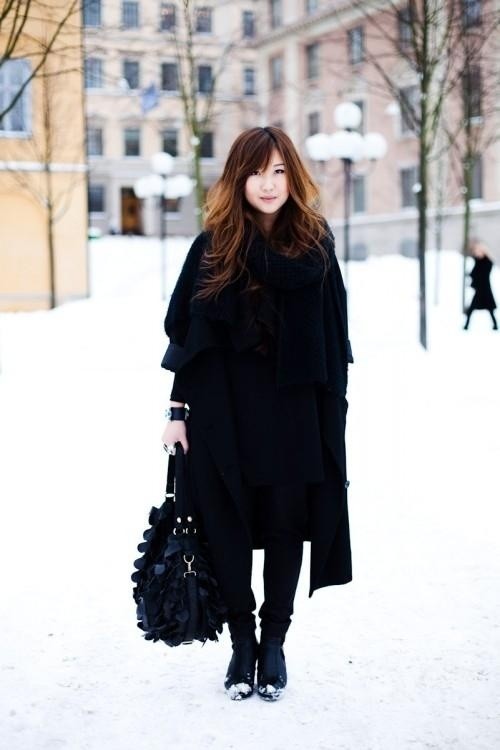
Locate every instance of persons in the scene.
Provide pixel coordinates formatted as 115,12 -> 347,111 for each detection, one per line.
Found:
154,126 -> 355,701
463,239 -> 498,330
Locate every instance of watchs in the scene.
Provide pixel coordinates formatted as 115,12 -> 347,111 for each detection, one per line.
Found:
163,406 -> 187,421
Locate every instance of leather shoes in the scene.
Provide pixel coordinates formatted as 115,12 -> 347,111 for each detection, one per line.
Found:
222,635 -> 294,704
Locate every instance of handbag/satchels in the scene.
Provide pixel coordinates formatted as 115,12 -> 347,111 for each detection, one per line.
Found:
132,440 -> 226,652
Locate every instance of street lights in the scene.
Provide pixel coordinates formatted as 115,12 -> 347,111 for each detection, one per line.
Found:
305,102 -> 385,260
134,154 -> 194,298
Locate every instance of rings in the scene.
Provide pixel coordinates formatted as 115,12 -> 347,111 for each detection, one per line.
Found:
163,445 -> 173,455
